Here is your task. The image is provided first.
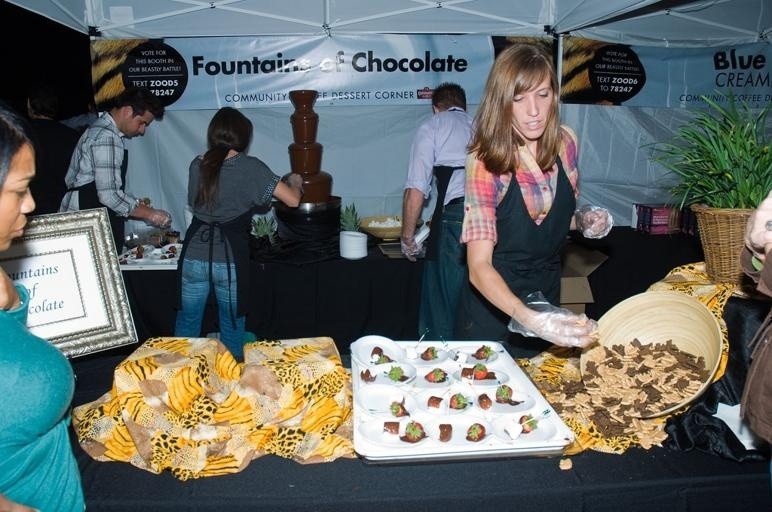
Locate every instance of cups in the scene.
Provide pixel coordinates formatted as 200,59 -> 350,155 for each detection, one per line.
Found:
125,228 -> 181,247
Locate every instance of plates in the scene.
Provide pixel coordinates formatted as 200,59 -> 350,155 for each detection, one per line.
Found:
117,243 -> 182,267
350,333 -> 559,447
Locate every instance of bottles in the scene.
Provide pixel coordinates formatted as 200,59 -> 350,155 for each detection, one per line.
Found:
282,90 -> 334,201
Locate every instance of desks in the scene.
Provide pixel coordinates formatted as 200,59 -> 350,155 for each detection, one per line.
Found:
116,227 -> 706,334
71,386 -> 772,512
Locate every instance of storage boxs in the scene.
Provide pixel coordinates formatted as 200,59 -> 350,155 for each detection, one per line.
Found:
630,202 -> 679,234
557,246 -> 610,314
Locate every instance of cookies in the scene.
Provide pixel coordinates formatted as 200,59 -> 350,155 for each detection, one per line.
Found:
535,338 -> 711,450
559,458 -> 573,470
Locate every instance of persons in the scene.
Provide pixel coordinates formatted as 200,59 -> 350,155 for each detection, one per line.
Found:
0,105 -> 89,512
738,189 -> 772,444
56,87 -> 173,255
400,80 -> 477,341
174,106 -> 305,364
416,39 -> 618,358
23,76 -> 77,216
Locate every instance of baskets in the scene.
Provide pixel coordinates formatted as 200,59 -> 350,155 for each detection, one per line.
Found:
689,203 -> 759,285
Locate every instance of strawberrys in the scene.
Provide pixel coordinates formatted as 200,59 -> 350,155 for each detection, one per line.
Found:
474,348 -> 486,359
426,372 -> 436,382
450,394 -> 464,409
496,385 -> 512,401
467,423 -> 485,442
473,364 -> 488,380
421,347 -> 432,360
395,404 -> 409,417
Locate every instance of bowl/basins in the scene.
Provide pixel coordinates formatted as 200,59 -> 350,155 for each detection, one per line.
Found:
578,287 -> 727,421
355,213 -> 426,244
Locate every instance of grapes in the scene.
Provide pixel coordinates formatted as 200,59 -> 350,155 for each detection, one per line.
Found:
406,423 -> 424,442
520,415 -> 532,433
